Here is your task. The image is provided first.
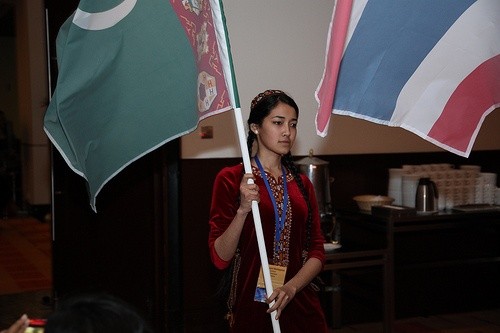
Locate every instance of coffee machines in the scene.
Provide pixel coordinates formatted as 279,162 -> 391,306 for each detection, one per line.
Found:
292,148 -> 338,244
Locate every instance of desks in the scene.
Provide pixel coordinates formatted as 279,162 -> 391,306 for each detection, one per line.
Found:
321,206 -> 500,333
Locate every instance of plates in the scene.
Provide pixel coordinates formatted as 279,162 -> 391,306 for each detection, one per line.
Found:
453,201 -> 499,213
386,164 -> 500,209
325,244 -> 341,251
354,195 -> 395,210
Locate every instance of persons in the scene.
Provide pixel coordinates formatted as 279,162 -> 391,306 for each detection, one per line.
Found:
207,88 -> 329,333
0,293 -> 157,333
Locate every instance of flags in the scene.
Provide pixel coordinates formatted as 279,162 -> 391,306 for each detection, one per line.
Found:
41,0 -> 240,215
313,0 -> 500,160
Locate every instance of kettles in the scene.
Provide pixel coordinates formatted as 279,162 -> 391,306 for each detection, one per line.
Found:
415,177 -> 439,213
